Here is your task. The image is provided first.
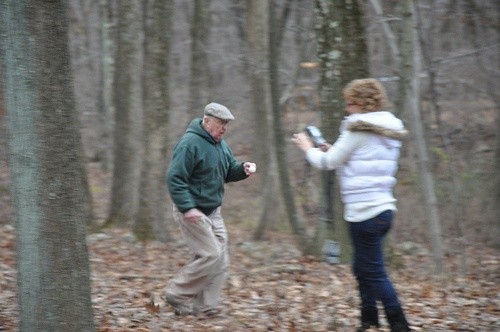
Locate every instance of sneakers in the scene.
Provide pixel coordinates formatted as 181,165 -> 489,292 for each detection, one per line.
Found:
160,291 -> 198,315
192,306 -> 229,319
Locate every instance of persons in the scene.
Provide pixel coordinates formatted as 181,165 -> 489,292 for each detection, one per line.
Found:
290,79 -> 411,332
158,102 -> 256,320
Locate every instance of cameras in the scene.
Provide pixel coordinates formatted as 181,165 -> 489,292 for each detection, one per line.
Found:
305,125 -> 327,148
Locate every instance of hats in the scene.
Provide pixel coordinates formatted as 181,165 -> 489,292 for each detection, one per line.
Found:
204,102 -> 234,120
